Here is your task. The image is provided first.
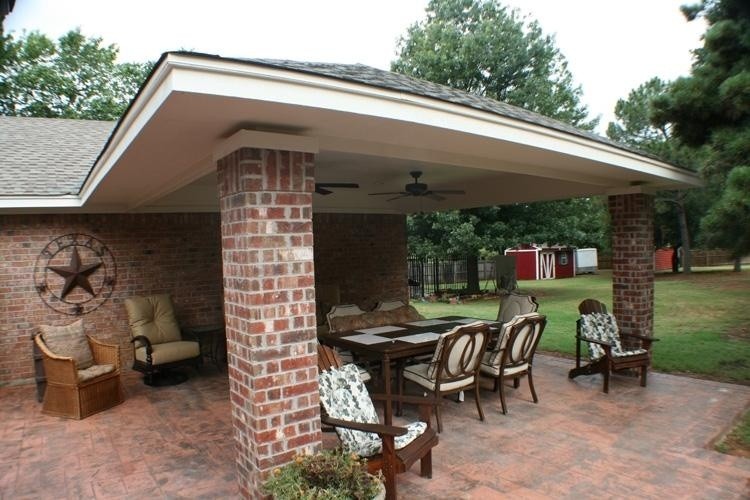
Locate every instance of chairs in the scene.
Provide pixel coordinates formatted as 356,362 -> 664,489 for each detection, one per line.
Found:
318,340 -> 372,383
34,332 -> 124,420
396,321 -> 491,433
317,344 -> 447,500
481,313 -> 547,414
569,298 -> 660,393
326,304 -> 380,371
493,294 -> 539,392
372,300 -> 405,311
125,294 -> 201,386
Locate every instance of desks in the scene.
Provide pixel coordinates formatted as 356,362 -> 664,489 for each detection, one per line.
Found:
189,324 -> 226,376
318,316 -> 503,416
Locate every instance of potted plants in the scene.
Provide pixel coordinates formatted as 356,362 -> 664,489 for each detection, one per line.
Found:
259,445 -> 386,500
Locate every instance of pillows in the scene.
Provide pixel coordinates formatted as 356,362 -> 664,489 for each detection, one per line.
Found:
40,319 -> 96,370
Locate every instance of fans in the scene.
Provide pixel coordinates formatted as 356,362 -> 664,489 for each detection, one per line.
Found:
368,171 -> 465,202
315,183 -> 359,194
